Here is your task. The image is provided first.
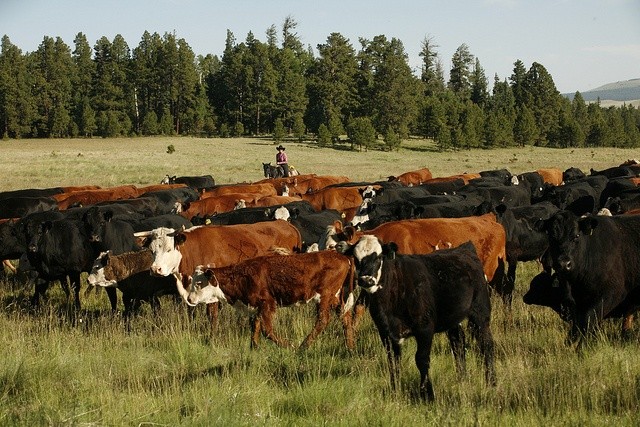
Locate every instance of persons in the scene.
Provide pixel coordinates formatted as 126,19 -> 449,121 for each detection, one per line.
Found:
275,144 -> 289,177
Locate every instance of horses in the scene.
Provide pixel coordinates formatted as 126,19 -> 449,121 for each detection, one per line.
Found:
262,161 -> 300,179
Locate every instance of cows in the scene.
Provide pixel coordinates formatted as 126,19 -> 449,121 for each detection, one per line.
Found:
132,219 -> 302,323
293,186 -> 363,213
386,195 -> 488,220
263,208 -> 347,253
190,200 -> 316,226
305,181 -> 373,195
0,188 -> 64,197
619,158 -> 640,173
521,210 -> 640,352
359,197 -> 416,220
358,184 -> 429,201
606,174 -> 640,195
535,167 -> 563,186
470,201 -> 562,310
197,183 -> 280,201
187,249 -> 357,357
448,173 -> 482,185
590,165 -> 636,180
278,175 -> 352,199
168,194 -> 262,219
387,168 -> 432,186
505,171 -> 544,189
237,173 -> 317,196
597,187 -> 640,216
561,167 -> 585,184
336,234 -> 497,408
61,185 -> 102,193
418,174 -> 470,187
137,183 -> 190,197
0,222 -> 23,285
421,178 -> 465,195
0,196 -> 58,219
74,184 -> 138,199
442,185 -> 532,207
333,212 -> 509,284
536,181 -> 600,217
569,174 -> 609,196
372,181 -> 405,188
233,195 -> 304,211
87,247 -> 184,336
85,206 -> 195,311
540,241 -> 562,288
52,189 -> 120,203
16,211 -> 95,315
478,168 -> 511,181
161,175 -> 215,190
58,190 -> 118,210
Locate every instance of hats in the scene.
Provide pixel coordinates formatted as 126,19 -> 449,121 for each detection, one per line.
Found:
276,145 -> 285,151
